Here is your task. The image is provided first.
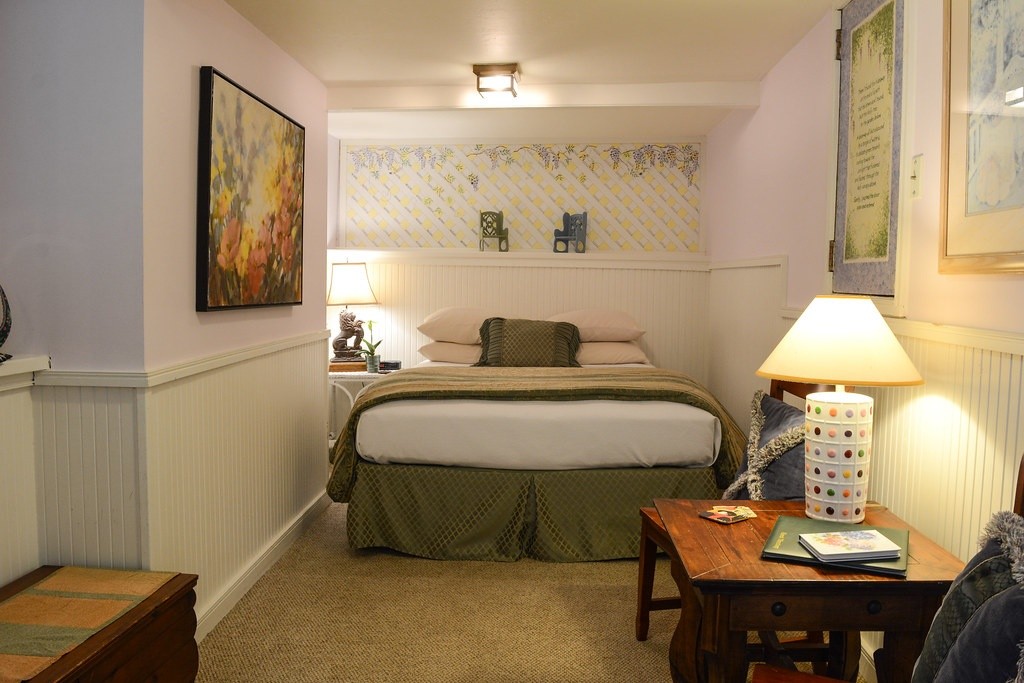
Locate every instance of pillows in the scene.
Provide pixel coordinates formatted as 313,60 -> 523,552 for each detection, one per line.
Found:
721,389 -> 805,500
416,310 -> 648,368
911,512 -> 1024,683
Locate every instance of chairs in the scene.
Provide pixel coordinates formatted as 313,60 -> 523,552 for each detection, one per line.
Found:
636,380 -> 852,642
477,210 -> 509,252
553,211 -> 587,253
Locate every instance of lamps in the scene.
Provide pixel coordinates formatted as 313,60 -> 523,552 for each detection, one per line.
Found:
474,63 -> 521,99
326,261 -> 377,363
755,295 -> 922,525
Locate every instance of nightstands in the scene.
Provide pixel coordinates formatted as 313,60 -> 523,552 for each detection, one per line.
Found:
328,371 -> 397,449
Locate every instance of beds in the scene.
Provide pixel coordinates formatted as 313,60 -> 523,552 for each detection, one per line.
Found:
326,356 -> 749,562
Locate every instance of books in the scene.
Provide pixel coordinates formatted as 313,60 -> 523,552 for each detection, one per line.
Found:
799,530 -> 901,562
762,515 -> 909,576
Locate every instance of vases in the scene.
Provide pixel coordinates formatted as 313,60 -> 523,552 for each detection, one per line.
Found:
365,355 -> 379,373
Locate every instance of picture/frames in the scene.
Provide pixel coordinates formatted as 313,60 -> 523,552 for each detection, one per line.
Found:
195,66 -> 306,312
939,1 -> 1023,275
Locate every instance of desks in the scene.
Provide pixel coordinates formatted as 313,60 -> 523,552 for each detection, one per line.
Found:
0,565 -> 199,683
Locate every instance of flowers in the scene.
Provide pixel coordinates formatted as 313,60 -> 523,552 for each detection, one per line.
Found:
353,320 -> 382,356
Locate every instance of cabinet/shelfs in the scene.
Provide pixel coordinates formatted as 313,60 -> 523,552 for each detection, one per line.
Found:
655,498 -> 967,683
